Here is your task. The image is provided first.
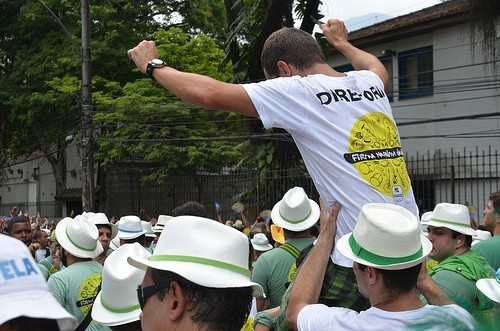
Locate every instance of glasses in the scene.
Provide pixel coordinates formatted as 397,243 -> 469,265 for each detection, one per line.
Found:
136,281 -> 183,311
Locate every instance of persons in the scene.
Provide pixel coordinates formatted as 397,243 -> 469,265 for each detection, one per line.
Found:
0,183 -> 500,331
127,19 -> 420,331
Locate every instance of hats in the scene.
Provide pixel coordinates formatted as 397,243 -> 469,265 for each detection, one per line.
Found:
91,242 -> 154,326
271,187 -> 320,232
420,202 -> 478,236
475,268 -> 500,303
127,214 -> 267,298
250,233 -> 273,252
140,221 -> 156,238
0,232 -> 77,330
336,202 -> 433,270
88,212 -> 118,240
54,214 -> 104,259
153,214 -> 172,232
421,211 -> 432,225
472,229 -> 491,247
115,215 -> 147,240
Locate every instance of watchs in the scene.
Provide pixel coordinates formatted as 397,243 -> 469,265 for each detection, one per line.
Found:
146,59 -> 167,81
